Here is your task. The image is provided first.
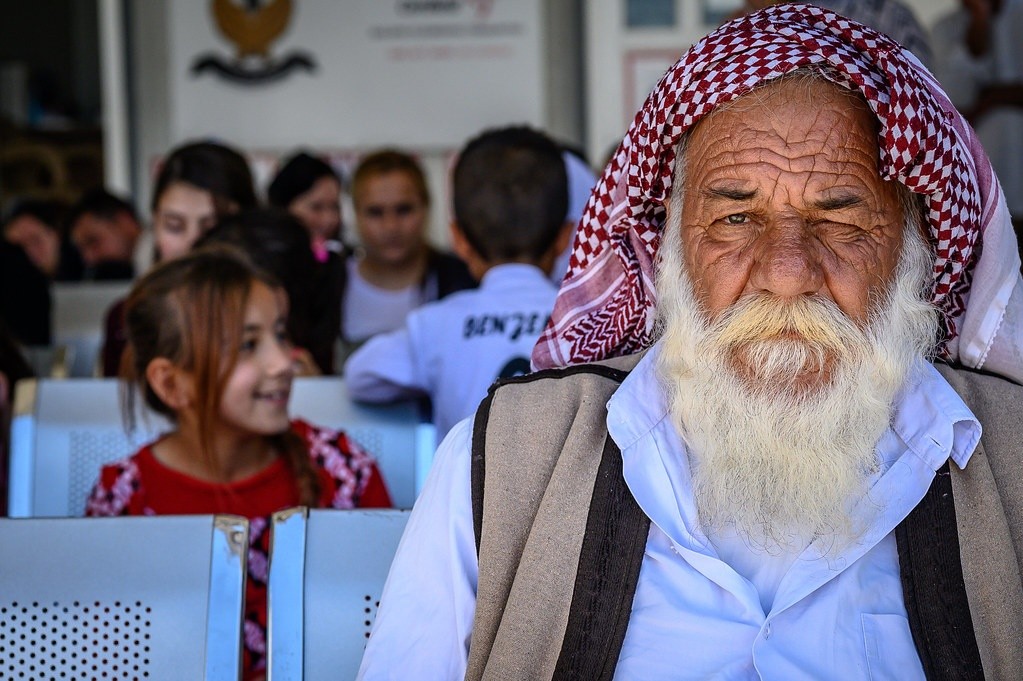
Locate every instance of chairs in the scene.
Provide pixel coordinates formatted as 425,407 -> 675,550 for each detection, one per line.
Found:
290,379 -> 420,511
0,514 -> 248,681
52,281 -> 132,368
265,509 -> 411,681
9,378 -> 177,516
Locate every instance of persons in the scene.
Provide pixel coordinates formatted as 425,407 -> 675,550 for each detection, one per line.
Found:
84,246 -> 395,681
0,125 -> 600,424
354,4 -> 1023,681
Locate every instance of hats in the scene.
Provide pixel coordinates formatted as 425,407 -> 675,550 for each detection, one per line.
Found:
531,2 -> 1022,382
